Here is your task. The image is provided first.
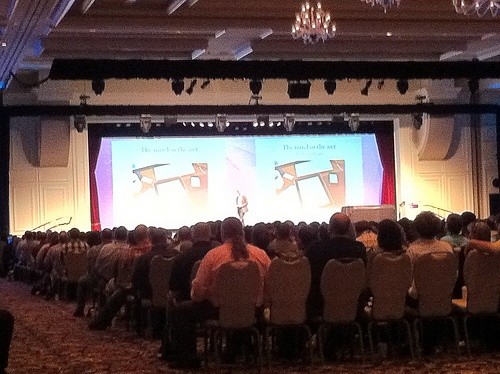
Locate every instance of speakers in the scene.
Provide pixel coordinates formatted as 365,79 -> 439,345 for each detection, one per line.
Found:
489,194 -> 500,216
416,115 -> 456,161
36,116 -> 71,167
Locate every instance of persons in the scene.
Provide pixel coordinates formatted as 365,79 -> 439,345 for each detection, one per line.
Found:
167,217 -> 272,369
0,212 -> 500,370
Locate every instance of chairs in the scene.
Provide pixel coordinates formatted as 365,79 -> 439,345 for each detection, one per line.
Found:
10,251 -> 500,367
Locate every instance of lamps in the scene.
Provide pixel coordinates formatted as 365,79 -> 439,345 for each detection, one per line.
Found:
361,0 -> 401,14
171,79 -> 184,94
91,78 -> 105,94
216,114 -> 228,132
453,0 -> 500,17
348,114 -> 359,133
411,113 -> 422,129
292,0 -> 336,44
467,79 -> 479,92
139,115 -> 152,133
283,113 -> 295,132
74,115 -> 86,132
396,79 -> 409,94
324,80 -> 336,94
250,79 -> 261,94
